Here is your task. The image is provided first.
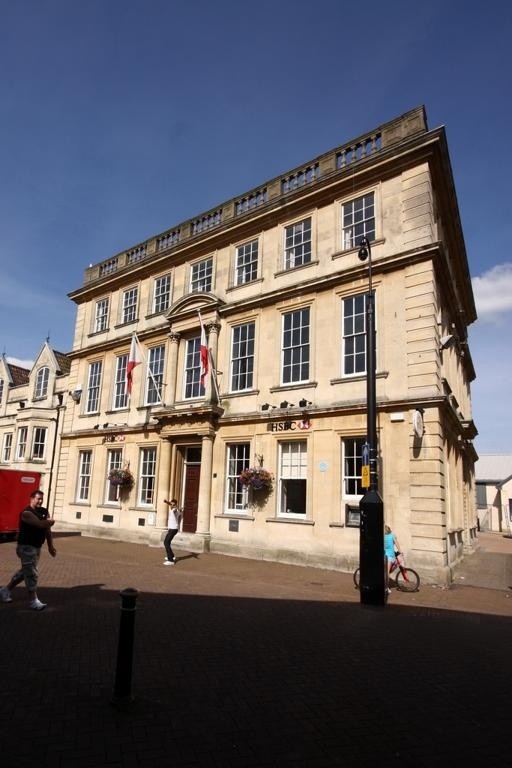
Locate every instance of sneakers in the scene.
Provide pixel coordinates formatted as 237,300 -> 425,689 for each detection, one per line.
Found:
163,556 -> 176,565
28,598 -> 47,611
0,587 -> 13,603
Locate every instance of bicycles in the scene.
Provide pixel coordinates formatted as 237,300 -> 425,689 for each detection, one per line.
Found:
354,552 -> 423,594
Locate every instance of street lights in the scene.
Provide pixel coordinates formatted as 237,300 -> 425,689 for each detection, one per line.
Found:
358,237 -> 376,491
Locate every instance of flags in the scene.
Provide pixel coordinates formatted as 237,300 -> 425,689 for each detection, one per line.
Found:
125,336 -> 142,396
200,327 -> 208,385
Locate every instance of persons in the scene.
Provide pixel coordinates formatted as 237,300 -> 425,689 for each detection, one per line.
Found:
163,498 -> 181,567
0,491 -> 56,610
384,525 -> 403,594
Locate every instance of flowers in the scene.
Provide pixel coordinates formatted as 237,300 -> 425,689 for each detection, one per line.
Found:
239,467 -> 274,496
108,468 -> 136,491
